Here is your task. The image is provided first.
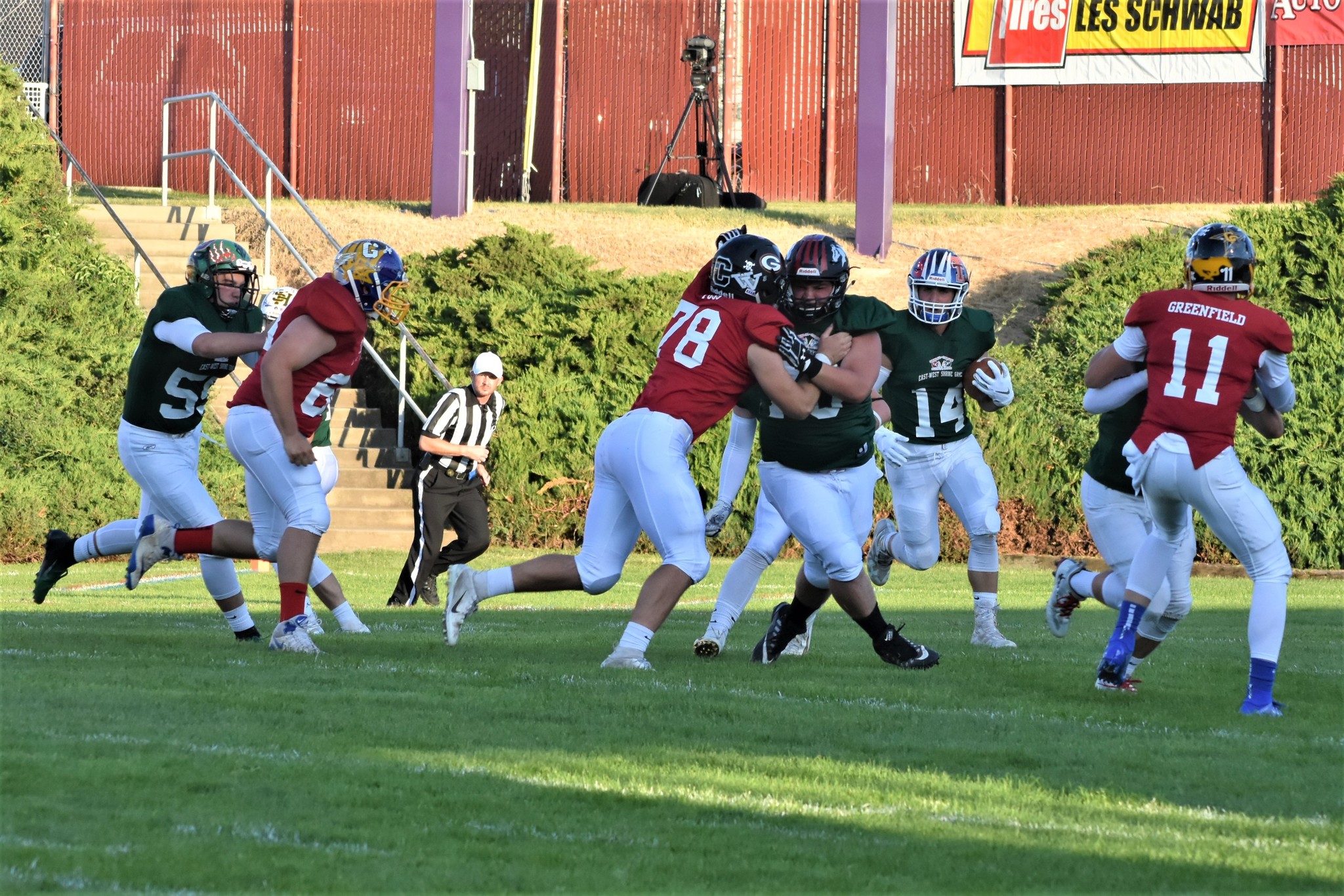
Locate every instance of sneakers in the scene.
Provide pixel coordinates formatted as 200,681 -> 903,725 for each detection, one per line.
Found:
269,614 -> 322,653
335,625 -> 371,633
601,641 -> 655,670
872,623 -> 940,671
694,638 -> 720,656
1095,671 -> 1142,692
420,575 -> 440,606
32,528 -> 72,604
750,601 -> 807,664
1046,557 -> 1087,639
866,519 -> 897,586
971,600 -> 1017,649
1238,699 -> 1285,716
442,563 -> 478,646
780,614 -> 816,655
124,514 -> 180,591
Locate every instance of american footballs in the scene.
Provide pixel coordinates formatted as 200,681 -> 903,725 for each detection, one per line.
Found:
963,357 -> 1003,400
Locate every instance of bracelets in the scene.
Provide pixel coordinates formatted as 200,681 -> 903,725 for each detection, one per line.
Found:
873,410 -> 882,429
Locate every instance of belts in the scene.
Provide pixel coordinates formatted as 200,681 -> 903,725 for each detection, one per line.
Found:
430,460 -> 469,480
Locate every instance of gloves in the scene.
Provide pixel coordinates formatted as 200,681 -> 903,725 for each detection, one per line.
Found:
971,360 -> 1014,407
715,225 -> 747,250
775,325 -> 823,379
704,500 -> 732,538
873,425 -> 910,470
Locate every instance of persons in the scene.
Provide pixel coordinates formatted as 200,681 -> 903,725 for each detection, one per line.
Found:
33,239 -> 277,643
693,383 -> 892,657
442,234 -> 852,670
866,248 -> 1017,648
386,351 -> 505,606
124,239 -> 409,654
715,224 -> 940,671
1044,352 -> 1284,693
1084,223 -> 1296,717
261,286 -> 371,633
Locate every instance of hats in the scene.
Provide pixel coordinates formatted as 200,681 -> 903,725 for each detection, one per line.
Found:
472,351 -> 503,379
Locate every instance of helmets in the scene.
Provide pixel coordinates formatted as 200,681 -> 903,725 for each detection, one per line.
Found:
185,239 -> 257,298
261,286 -> 299,331
781,235 -> 850,309
1181,223 -> 1257,301
710,235 -> 785,306
333,239 -> 404,310
907,248 -> 969,310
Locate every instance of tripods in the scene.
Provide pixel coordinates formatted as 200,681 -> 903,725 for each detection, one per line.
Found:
643,68 -> 738,209
